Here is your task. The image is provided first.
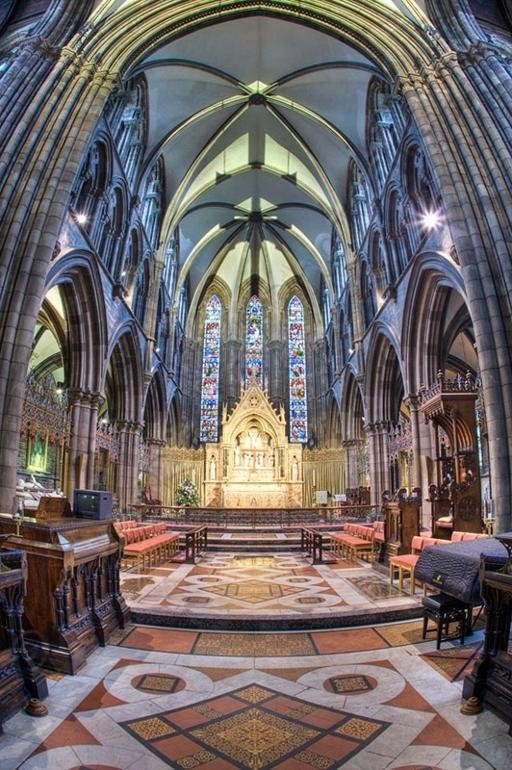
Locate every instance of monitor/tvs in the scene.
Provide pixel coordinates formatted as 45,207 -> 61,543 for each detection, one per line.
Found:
73,488 -> 113,520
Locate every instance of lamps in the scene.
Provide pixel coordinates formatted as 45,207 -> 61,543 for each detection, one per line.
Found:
120,283 -> 130,298
348,339 -> 362,353
378,286 -> 392,303
154,342 -> 161,354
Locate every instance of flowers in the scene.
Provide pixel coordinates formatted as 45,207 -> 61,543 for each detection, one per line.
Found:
175,479 -> 202,507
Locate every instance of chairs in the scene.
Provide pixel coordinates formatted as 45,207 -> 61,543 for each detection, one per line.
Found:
330,520 -> 491,651
142,490 -> 162,516
113,520 -> 179,575
